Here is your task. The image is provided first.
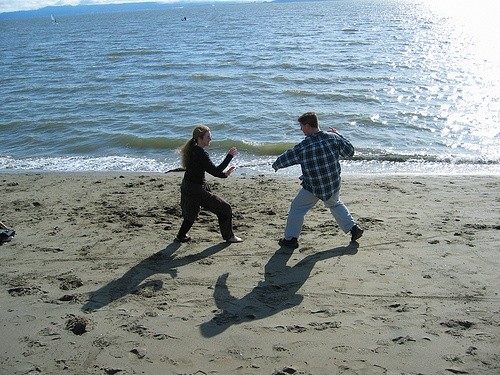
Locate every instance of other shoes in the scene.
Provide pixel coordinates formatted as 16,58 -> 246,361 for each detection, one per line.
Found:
177,235 -> 191,242
349,224 -> 363,241
226,235 -> 243,242
278,237 -> 299,249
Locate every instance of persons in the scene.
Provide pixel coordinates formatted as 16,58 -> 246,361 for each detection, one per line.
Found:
272,112 -> 364,248
173,125 -> 243,243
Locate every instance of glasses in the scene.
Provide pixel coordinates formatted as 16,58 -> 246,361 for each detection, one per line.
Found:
202,135 -> 212,139
